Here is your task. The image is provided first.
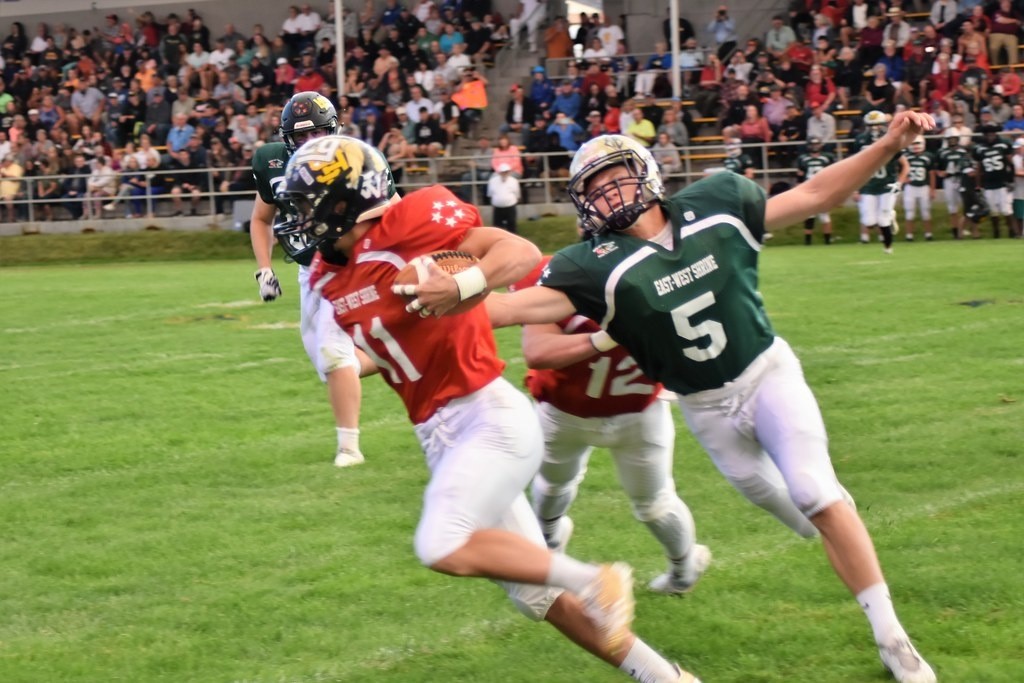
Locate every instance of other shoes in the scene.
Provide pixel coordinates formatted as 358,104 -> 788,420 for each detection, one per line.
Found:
550,514 -> 573,553
654,660 -> 700,683
585,559 -> 634,651
649,544 -> 712,594
883,246 -> 892,254
877,636 -> 937,683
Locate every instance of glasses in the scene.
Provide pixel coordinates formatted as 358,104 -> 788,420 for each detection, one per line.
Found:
809,141 -> 819,144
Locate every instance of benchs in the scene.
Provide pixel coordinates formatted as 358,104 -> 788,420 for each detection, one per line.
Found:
18,27 -> 515,203
494,9 -> 1024,173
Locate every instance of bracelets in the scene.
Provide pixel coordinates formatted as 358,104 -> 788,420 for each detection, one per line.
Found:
452,266 -> 487,301
590,330 -> 619,353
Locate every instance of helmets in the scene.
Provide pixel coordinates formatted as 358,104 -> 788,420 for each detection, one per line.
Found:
275,135 -> 402,264
908,133 -> 925,152
569,134 -> 662,236
278,91 -> 338,155
864,110 -> 886,124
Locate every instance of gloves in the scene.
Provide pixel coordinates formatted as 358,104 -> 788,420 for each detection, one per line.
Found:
254,268 -> 281,299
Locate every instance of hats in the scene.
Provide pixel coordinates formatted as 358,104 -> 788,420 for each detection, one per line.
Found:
394,106 -> 405,114
809,101 -> 819,108
589,110 -> 601,116
973,121 -> 1002,131
932,101 -> 939,109
497,163 -> 511,172
941,127 -> 960,138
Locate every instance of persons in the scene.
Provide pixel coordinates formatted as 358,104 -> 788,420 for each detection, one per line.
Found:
0,0 -> 1024,255
250,91 -> 937,683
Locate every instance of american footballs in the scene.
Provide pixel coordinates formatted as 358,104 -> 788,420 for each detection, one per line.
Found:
392,250 -> 491,317
